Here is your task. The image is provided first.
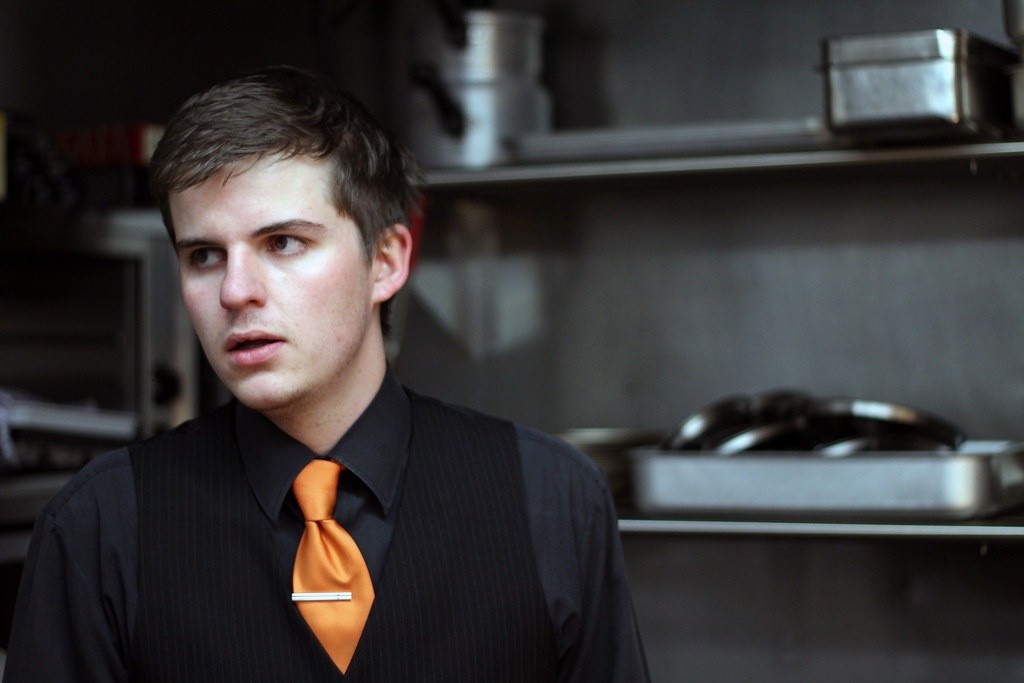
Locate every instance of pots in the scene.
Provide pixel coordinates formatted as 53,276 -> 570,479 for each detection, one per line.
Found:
406,0 -> 555,170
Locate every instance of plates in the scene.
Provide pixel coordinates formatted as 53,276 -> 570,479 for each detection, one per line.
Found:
661,386 -> 965,453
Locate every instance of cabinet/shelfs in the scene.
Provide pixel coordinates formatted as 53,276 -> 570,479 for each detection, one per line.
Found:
379,121 -> 1024,544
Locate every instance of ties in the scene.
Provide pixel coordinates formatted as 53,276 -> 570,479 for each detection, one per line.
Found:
290,458 -> 375,673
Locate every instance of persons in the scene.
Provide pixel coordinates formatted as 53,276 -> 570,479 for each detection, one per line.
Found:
2,65 -> 653,683
